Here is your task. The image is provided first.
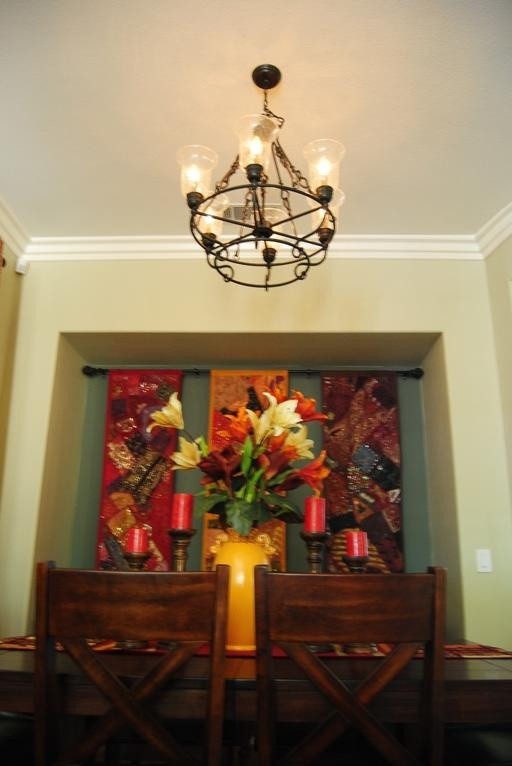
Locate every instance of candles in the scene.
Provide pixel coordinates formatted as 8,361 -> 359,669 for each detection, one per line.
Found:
128,526 -> 149,554
305,496 -> 327,532
346,530 -> 368,557
170,493 -> 193,531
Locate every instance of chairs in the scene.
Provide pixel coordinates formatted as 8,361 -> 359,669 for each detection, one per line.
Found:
251,565 -> 448,765
31,561 -> 231,764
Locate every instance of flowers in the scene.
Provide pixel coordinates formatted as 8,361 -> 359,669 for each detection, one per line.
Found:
145,382 -> 333,532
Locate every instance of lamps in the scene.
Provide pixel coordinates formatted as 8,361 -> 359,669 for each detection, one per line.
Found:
180,67 -> 344,290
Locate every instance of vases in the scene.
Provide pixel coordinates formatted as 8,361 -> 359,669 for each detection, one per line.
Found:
211,531 -> 277,653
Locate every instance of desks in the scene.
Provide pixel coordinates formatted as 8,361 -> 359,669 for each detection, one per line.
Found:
1,639 -> 511,730
